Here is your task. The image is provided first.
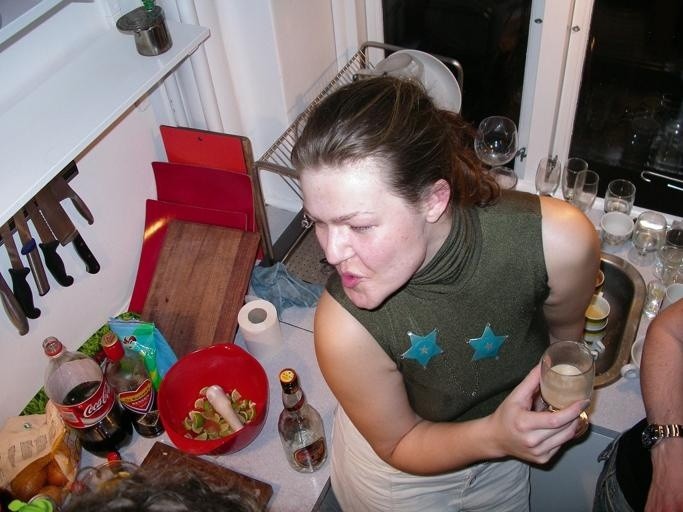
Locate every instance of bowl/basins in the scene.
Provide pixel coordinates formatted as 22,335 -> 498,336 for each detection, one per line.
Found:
156,342 -> 270,456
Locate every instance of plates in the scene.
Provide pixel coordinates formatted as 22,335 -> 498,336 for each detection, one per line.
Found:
384,48 -> 462,119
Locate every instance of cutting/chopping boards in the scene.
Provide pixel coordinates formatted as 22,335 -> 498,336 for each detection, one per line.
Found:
140,219 -> 261,361
122,441 -> 273,511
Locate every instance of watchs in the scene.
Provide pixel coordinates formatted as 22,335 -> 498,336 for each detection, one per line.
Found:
640,422 -> 683,450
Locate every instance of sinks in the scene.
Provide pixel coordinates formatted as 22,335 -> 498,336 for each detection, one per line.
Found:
593,249 -> 646,391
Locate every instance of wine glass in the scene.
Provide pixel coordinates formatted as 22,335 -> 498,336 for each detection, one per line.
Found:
538,340 -> 595,440
474,116 -> 667,267
386,54 -> 423,82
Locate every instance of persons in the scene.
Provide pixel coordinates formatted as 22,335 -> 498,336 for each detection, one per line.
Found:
591,298 -> 683,511
289,76 -> 602,511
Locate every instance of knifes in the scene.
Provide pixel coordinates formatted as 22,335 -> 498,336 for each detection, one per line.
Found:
0,174 -> 101,336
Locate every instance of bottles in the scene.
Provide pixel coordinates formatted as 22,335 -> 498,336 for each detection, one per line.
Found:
278,368 -> 328,474
43,332 -> 165,458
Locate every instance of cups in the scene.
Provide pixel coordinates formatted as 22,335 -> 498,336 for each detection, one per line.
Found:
585,211 -> 683,382
